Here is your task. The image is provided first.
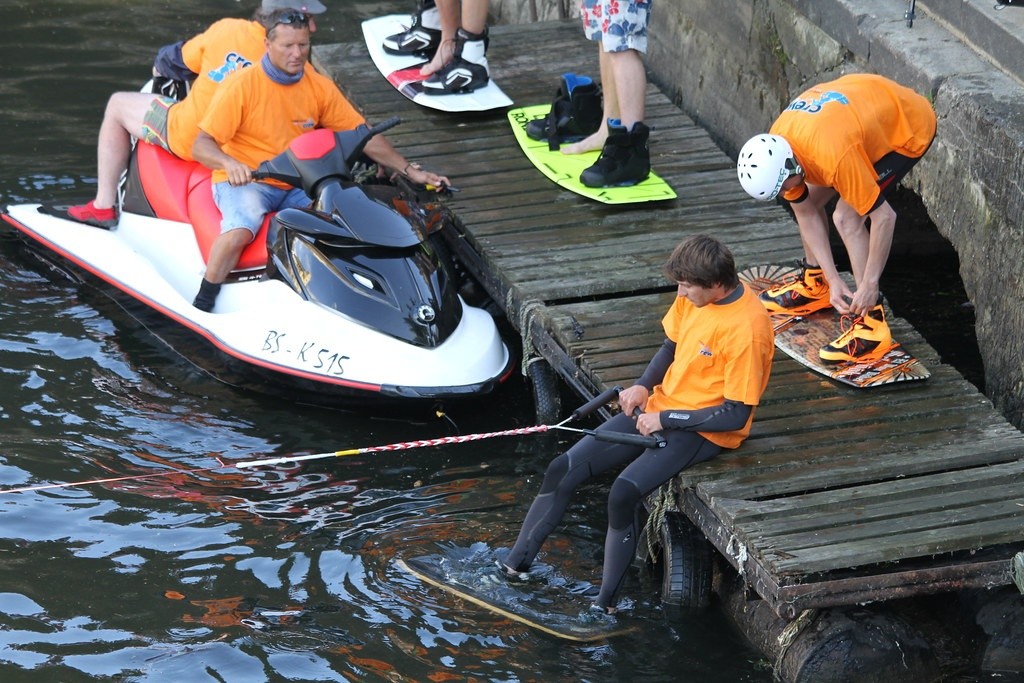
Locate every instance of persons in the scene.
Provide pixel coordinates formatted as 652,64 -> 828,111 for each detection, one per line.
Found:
420,0 -> 490,93
193,9 -> 451,312
68,1 -> 325,229
446,235 -> 775,632
559,0 -> 652,187
737,73 -> 938,364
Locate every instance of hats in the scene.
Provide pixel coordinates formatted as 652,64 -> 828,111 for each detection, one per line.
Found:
262,0 -> 326,15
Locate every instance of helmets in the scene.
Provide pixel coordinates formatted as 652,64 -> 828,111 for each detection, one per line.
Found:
737,133 -> 803,202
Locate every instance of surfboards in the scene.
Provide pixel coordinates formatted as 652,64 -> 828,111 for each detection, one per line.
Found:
736,263 -> 934,391
360,13 -> 515,114
400,553 -> 641,644
508,103 -> 680,206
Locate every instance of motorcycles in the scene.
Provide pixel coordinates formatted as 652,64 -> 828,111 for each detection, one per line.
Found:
0,117 -> 521,437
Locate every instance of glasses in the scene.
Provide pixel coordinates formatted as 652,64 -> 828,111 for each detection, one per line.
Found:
265,13 -> 307,36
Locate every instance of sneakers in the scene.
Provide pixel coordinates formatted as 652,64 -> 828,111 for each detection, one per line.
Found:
382,2 -> 442,56
819,304 -> 892,366
579,119 -> 651,188
758,259 -> 833,315
570,603 -> 620,632
475,559 -> 529,587
524,74 -> 603,150
422,25 -> 491,95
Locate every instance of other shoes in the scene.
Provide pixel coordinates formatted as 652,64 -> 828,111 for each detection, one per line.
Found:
67,199 -> 116,227
